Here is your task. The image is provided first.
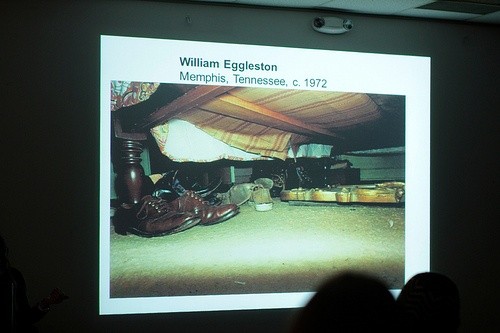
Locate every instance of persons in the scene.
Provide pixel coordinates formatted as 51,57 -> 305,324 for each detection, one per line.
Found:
287,272 -> 397,333
0,237 -> 70,333
397,272 -> 458,333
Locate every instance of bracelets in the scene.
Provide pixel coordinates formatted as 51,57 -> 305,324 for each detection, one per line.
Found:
38,303 -> 50,313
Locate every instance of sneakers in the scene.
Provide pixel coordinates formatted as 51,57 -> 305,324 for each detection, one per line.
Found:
220,178 -> 273,207
248,184 -> 273,212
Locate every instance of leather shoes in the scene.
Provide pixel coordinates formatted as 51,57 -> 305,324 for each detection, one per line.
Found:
117,195 -> 202,238
288,157 -> 324,188
167,189 -> 240,225
267,165 -> 289,196
151,170 -> 224,205
